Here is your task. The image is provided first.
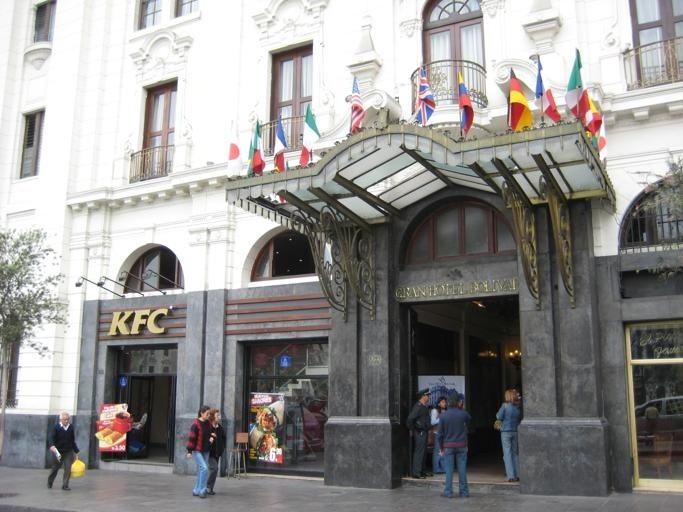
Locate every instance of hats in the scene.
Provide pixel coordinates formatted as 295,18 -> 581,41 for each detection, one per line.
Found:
416,388 -> 432,397
257,400 -> 285,427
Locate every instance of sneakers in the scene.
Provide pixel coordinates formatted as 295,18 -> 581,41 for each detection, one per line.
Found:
197,492 -> 205,497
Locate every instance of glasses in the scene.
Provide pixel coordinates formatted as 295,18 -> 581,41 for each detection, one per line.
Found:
58,417 -> 71,420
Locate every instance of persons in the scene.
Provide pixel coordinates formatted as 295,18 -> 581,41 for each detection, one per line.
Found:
645,404 -> 659,420
186,406 -> 215,499
438,395 -> 471,498
408,388 -> 433,479
207,408 -> 225,494
48,412 -> 80,490
431,397 -> 447,474
496,390 -> 520,482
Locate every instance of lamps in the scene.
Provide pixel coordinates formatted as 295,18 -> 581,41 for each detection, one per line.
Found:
117,269 -> 166,296
477,350 -> 497,365
141,268 -> 183,288
74,275 -> 125,298
509,349 -> 522,370
96,275 -> 143,297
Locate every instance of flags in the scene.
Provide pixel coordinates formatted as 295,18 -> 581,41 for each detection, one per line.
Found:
414,65 -> 435,124
591,116 -> 606,152
248,118 -> 266,176
300,104 -> 319,166
350,76 -> 366,133
566,48 -> 602,139
273,114 -> 287,172
457,71 -> 474,140
536,54 -> 561,125
507,66 -> 533,131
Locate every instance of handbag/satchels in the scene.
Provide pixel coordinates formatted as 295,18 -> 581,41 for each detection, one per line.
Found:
427,409 -> 440,447
493,403 -> 506,432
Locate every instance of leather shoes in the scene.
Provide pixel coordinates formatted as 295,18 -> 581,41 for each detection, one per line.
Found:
205,490 -> 213,494
423,471 -> 431,474
509,477 -> 519,481
61,484 -> 67,488
458,494 -> 468,497
47,482 -> 52,485
435,471 -> 442,474
440,491 -> 451,498
192,491 -> 197,495
442,471 -> 447,474
413,474 -> 426,478
194,490 -> 198,492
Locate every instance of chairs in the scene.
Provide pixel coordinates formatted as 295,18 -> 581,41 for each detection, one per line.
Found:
228,432 -> 248,480
639,431 -> 673,480
